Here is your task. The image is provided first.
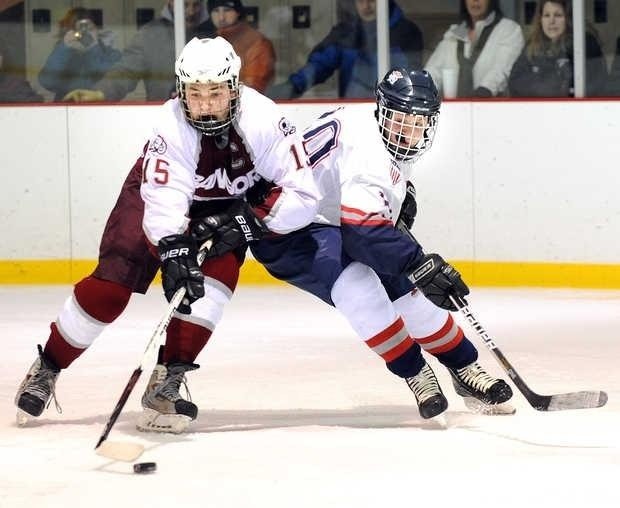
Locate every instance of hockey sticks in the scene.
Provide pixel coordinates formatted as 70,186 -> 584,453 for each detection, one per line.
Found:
91,240 -> 216,463
398,220 -> 608,412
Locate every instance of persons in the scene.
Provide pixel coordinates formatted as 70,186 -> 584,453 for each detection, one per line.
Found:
14,35 -> 324,433
251,65 -> 514,420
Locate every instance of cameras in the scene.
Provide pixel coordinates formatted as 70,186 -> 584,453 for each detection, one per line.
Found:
75,32 -> 93,46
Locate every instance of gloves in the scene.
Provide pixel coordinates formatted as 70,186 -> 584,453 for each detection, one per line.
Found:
397,179 -> 418,230
404,251 -> 468,310
192,208 -> 264,260
156,233 -> 206,315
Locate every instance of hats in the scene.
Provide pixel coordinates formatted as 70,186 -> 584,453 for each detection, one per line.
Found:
204,1 -> 244,17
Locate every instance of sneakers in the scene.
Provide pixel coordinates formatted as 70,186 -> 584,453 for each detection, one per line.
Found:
404,361 -> 448,419
14,343 -> 62,418
448,360 -> 514,405
140,343 -> 201,420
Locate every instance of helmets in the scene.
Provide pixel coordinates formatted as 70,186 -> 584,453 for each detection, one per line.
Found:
173,36 -> 243,134
374,66 -> 441,162
